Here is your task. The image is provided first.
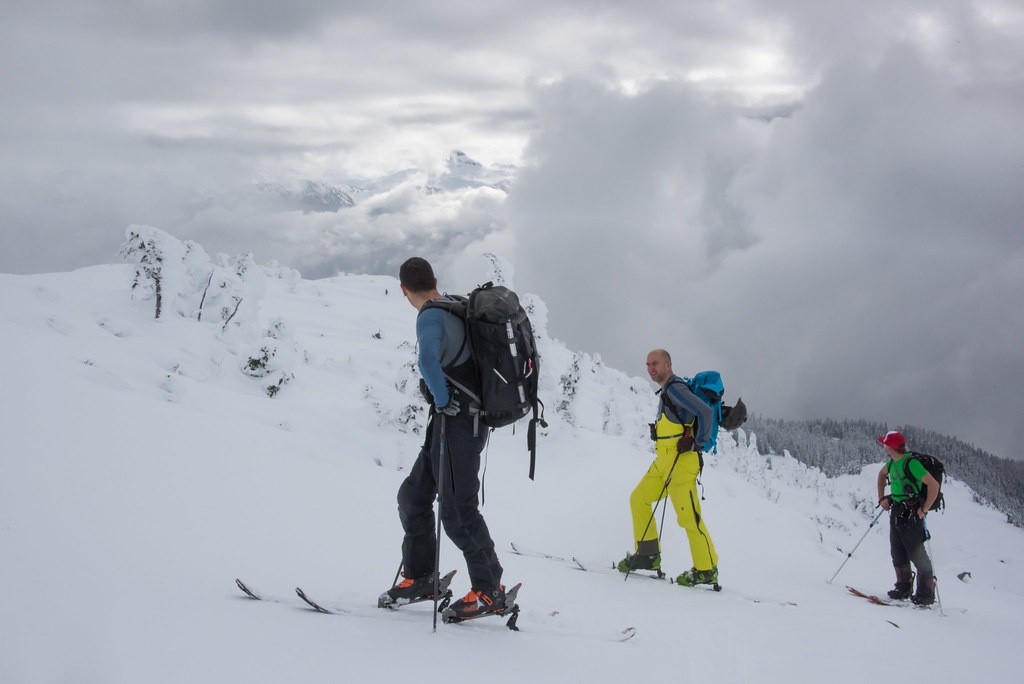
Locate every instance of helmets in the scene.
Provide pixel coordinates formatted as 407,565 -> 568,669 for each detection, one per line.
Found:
718,397 -> 748,431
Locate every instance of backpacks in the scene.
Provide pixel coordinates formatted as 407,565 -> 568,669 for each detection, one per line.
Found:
416,281 -> 541,428
660,370 -> 726,455
888,451 -> 947,512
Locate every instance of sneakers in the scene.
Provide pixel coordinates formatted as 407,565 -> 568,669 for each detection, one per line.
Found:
442,584 -> 506,623
676,568 -> 718,588
618,551 -> 662,571
378,571 -> 440,608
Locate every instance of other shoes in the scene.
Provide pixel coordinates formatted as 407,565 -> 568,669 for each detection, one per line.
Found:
888,580 -> 913,599
911,591 -> 934,606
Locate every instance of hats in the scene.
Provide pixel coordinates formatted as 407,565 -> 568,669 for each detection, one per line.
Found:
875,431 -> 906,450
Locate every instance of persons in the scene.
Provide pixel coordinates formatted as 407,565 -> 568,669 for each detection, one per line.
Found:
876,431 -> 940,606
617,348 -> 719,587
377,256 -> 522,624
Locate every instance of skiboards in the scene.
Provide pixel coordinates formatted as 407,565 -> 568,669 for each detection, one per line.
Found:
840,580 -> 938,610
504,541 -> 801,611
228,579 -> 638,646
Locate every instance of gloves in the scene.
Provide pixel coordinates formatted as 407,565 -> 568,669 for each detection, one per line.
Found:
436,395 -> 461,416
677,436 -> 694,455
